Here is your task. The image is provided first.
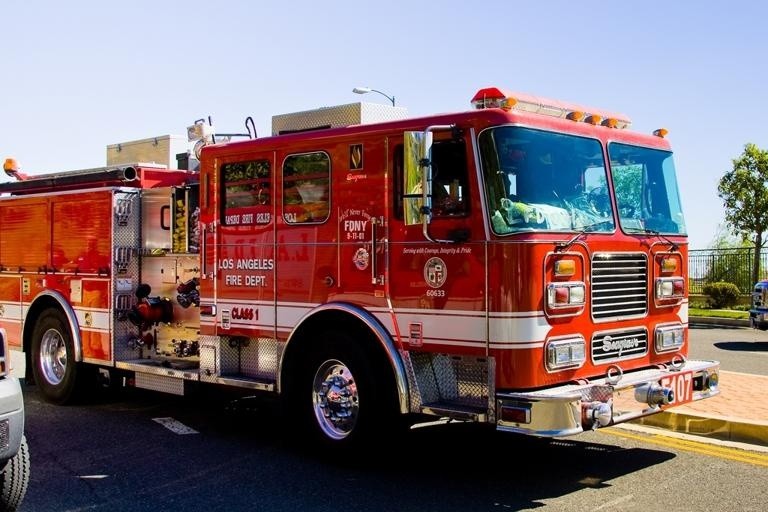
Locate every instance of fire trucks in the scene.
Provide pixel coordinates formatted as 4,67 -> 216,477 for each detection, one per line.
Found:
0,88 -> 719,471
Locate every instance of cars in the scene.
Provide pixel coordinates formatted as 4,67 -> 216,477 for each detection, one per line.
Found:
0,328 -> 31,512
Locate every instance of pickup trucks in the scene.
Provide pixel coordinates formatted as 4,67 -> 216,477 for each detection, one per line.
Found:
750,281 -> 768,332
